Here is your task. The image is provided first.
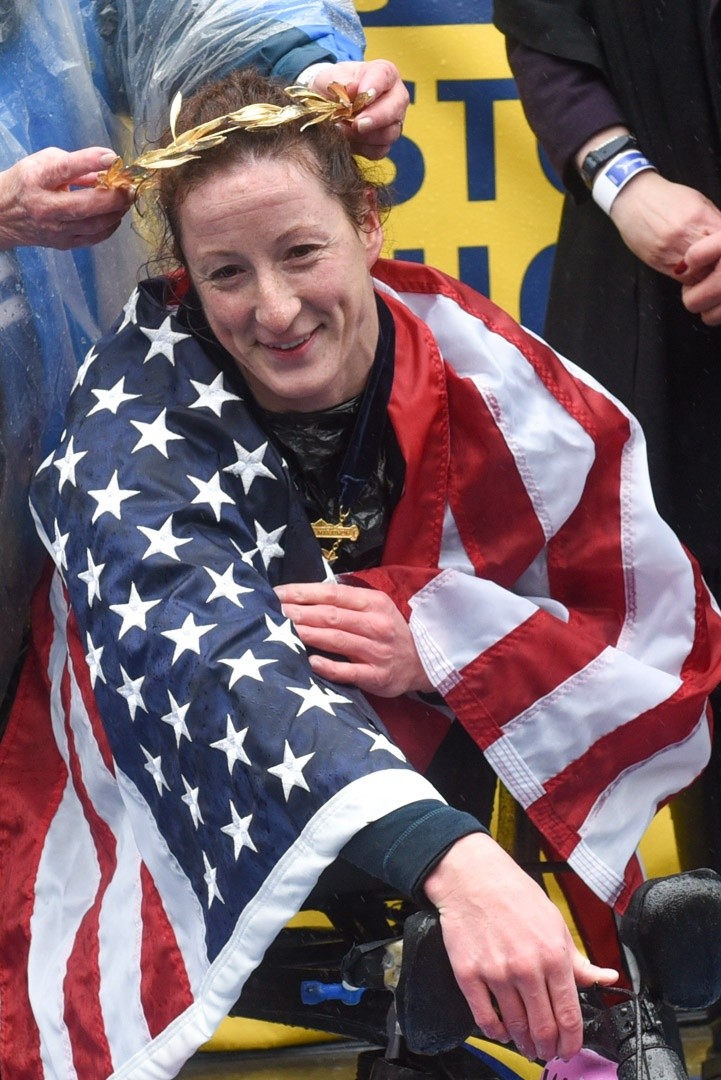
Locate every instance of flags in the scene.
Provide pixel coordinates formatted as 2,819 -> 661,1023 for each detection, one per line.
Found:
0,260 -> 721,1080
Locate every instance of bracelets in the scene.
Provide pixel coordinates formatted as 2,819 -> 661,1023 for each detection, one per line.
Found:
592,149 -> 657,216
581,136 -> 643,189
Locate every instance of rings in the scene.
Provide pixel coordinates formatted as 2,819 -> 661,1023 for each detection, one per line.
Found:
398,119 -> 403,135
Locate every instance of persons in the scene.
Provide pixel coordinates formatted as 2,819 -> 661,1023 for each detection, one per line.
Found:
57,73 -> 705,1080
491,0 -> 721,1080
0,0 -> 411,762
